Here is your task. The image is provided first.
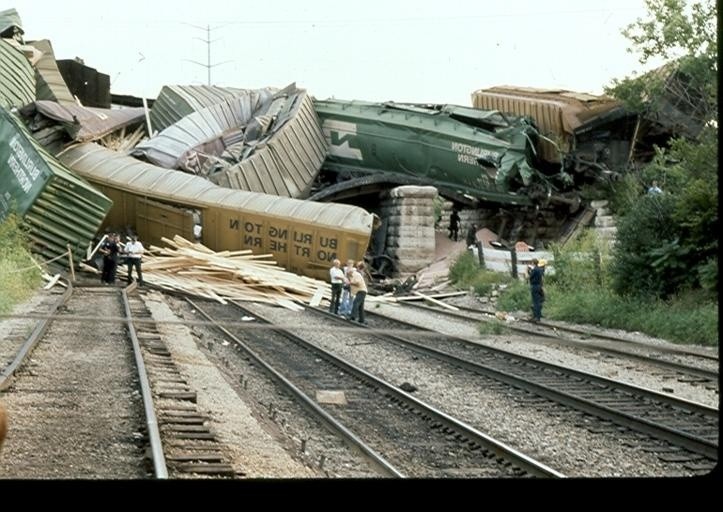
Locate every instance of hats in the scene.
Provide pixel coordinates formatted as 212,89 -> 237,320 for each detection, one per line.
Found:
537,259 -> 546,267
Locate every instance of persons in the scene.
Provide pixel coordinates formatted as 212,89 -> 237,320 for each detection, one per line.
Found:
649,179 -> 662,193
329,259 -> 347,314
526,258 -> 543,322
448,209 -> 460,241
336,260 -> 356,317
466,223 -> 478,253
98,232 -> 118,284
108,232 -> 123,286
353,261 -> 366,276
123,235 -> 145,288
346,266 -> 368,323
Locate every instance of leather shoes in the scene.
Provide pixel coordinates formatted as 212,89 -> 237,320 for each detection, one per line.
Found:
529,316 -> 540,322
